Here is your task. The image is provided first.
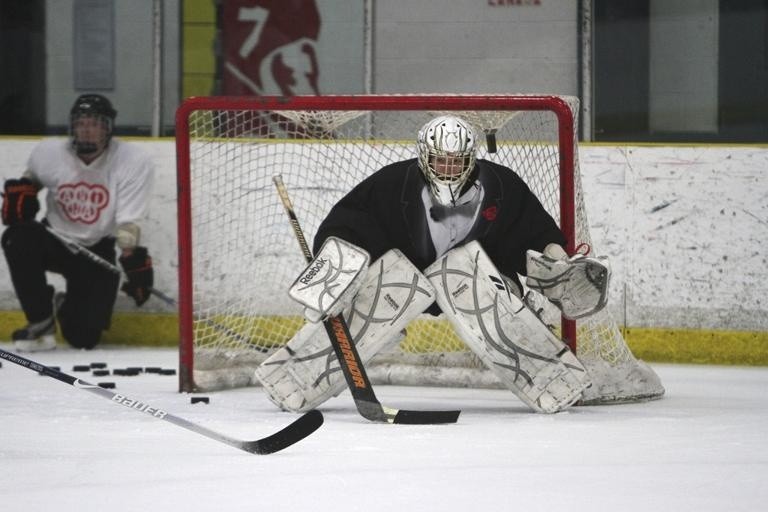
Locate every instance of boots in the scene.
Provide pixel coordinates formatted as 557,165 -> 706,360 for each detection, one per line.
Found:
11,318 -> 57,341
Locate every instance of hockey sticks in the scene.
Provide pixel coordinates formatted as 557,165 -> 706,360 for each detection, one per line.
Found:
0,348 -> 323,458
32,216 -> 281,355
273,174 -> 461,425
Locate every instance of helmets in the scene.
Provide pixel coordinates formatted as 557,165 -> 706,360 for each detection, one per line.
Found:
417,114 -> 477,208
70,94 -> 118,120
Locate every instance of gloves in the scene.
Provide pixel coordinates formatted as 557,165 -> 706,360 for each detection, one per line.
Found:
118,247 -> 154,307
2,177 -> 42,226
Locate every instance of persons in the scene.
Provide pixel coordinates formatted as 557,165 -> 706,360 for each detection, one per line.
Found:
0,93 -> 158,350
251,113 -> 610,414
